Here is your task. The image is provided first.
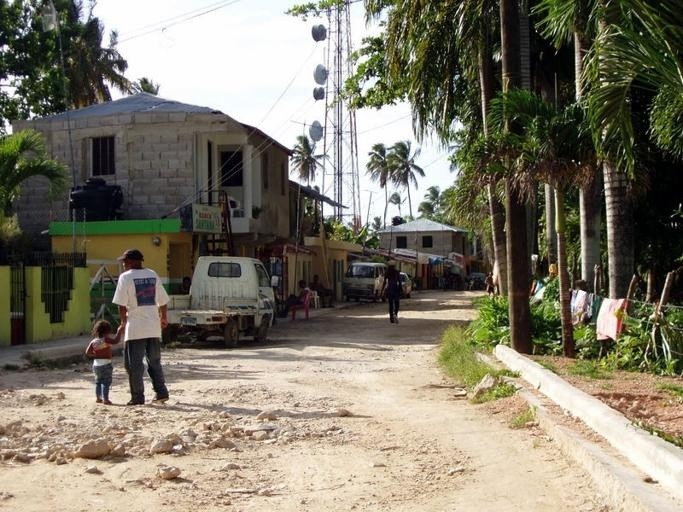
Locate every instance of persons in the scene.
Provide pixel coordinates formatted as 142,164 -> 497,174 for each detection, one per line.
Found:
486,272 -> 495,296
86,248 -> 170,406
308,275 -> 335,308
464,278 -> 483,290
380,260 -> 403,324
575,279 -> 592,326
280,280 -> 310,318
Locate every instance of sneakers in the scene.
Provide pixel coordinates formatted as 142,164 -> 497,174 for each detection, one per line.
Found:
152,395 -> 169,402
97,400 -> 112,404
393,315 -> 399,324
126,399 -> 145,406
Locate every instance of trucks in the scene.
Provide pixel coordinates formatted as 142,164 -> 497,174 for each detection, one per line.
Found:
159,255 -> 280,348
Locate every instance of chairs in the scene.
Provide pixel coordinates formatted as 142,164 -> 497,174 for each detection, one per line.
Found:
290,290 -> 321,320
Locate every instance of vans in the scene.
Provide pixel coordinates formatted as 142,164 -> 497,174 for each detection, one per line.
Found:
343,262 -> 388,303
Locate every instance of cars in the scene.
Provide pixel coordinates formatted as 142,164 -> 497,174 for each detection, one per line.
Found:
397,271 -> 412,299
467,273 -> 487,289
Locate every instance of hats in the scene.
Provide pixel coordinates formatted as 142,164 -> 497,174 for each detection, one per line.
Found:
388,260 -> 397,266
117,248 -> 144,261
